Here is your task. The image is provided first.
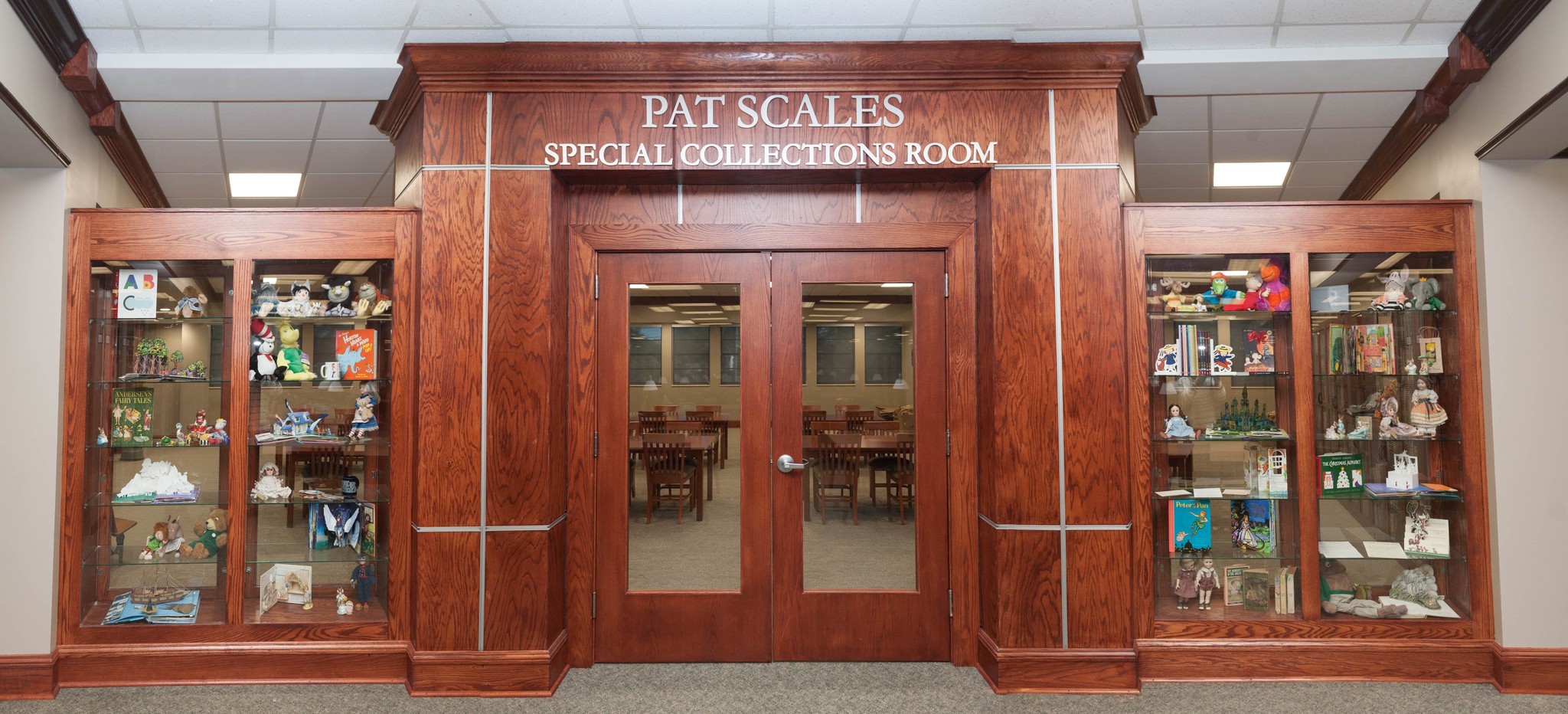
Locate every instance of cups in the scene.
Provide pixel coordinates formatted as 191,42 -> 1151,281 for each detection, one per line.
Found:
341,475 -> 359,496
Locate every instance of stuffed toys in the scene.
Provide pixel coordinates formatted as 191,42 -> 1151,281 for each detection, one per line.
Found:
174,286 -> 208,318
208,418 -> 229,445
356,397 -> 373,421
1319,558 -> 1407,618
251,277 -> 391,318
1158,261 -> 1290,311
139,506 -> 227,559
250,320 -> 317,381
1367,263 -> 1447,311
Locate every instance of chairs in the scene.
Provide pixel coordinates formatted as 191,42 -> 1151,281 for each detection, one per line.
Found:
292,407 -> 356,518
629,405 -> 916,525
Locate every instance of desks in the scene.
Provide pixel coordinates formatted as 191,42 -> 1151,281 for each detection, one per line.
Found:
630,415 -> 729,469
1168,445 -> 1194,490
628,436 -> 717,521
275,418 -> 365,528
802,436 -> 914,522
802,414 -> 886,426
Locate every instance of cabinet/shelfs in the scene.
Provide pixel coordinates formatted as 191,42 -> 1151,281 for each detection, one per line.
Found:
56,207 -> 421,688
1121,200 -> 1493,685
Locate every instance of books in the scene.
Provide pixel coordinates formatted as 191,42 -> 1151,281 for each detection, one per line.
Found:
113,268 -> 119,319
1179,324 -> 1215,375
1418,337 -> 1443,374
1377,595 -> 1462,619
1222,564 -> 1297,614
308,499 -> 376,558
1315,451 -> 1365,496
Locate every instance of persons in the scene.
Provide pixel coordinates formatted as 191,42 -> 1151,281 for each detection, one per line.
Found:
250,462 -> 291,500
190,409 -> 207,441
1379,375 -> 1448,439
1160,403 -> 1201,439
351,553 -> 376,612
344,380 -> 381,441
1174,553 -> 1220,610
